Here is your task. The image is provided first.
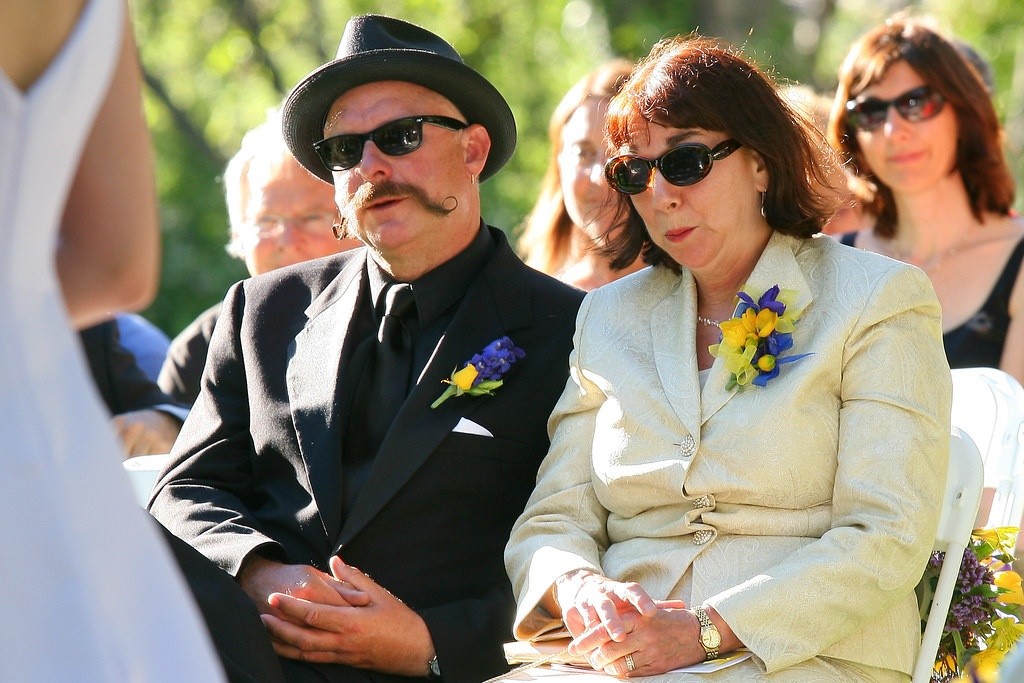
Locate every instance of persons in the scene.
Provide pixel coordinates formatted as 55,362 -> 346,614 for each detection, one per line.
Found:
0,0 -> 366,683
503,32 -> 953,683
151,10 -> 588,683
777,21 -> 1024,394
518,59 -> 672,291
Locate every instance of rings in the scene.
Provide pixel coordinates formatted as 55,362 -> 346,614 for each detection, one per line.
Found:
625,655 -> 635,674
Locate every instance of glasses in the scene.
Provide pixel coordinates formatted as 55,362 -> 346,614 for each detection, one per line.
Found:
252,213 -> 331,240
603,139 -> 740,195
847,83 -> 944,132
313,115 -> 465,171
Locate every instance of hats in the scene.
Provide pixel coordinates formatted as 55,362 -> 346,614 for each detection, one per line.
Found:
281,16 -> 516,188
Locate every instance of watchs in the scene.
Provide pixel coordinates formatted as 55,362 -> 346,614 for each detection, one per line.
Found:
427,652 -> 442,683
691,606 -> 721,664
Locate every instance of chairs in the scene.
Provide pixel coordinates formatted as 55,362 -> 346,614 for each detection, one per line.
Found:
913,366 -> 1024,683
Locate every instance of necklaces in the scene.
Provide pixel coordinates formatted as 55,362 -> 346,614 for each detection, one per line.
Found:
697,314 -> 733,343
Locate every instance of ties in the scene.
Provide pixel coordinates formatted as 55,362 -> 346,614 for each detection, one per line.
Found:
350,283 -> 414,463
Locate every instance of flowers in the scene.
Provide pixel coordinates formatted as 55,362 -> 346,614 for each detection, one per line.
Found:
709,283 -> 822,393
923,528 -> 1024,683
428,335 -> 525,407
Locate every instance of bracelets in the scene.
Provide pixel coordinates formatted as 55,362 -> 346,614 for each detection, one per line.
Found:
551,581 -> 564,623
